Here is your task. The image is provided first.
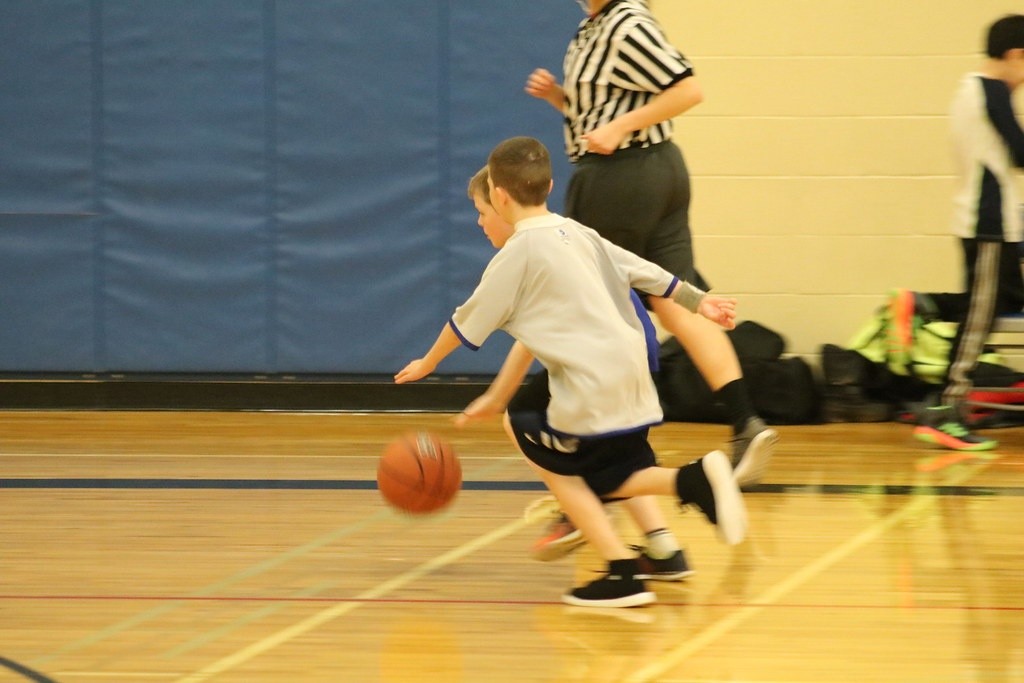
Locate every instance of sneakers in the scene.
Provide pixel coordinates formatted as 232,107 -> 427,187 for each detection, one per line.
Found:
529,509 -> 616,561
676,450 -> 746,545
890,291 -> 931,364
562,570 -> 657,608
729,415 -> 780,486
627,544 -> 693,581
913,404 -> 999,451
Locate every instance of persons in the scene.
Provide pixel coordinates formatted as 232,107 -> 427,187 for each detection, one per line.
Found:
394,136 -> 750,607
524,0 -> 778,487
911,16 -> 1024,450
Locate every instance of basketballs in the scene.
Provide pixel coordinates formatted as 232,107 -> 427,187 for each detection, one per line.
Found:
373,430 -> 465,514
